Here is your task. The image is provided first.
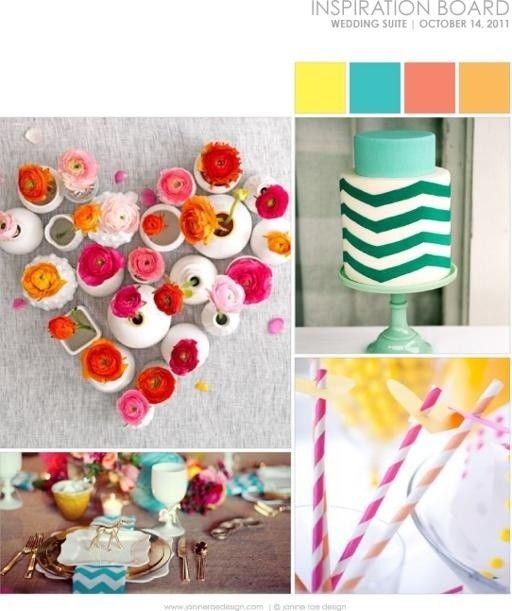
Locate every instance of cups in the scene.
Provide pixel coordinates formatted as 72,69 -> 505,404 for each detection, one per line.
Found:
53,480 -> 92,522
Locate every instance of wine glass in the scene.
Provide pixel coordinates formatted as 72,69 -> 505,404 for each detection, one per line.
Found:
152,464 -> 189,537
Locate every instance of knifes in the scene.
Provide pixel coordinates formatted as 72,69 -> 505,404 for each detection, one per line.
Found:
176,535 -> 190,583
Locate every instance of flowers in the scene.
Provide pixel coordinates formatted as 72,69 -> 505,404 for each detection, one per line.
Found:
33,451 -> 228,518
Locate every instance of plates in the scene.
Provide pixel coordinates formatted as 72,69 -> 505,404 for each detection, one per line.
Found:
35,526 -> 175,583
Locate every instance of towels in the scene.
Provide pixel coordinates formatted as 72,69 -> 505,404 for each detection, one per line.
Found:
71,516 -> 136,594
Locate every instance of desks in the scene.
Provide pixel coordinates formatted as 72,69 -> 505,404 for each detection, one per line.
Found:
294,325 -> 510,356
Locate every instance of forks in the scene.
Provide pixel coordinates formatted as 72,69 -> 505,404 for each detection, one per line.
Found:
0,532 -> 43,579
